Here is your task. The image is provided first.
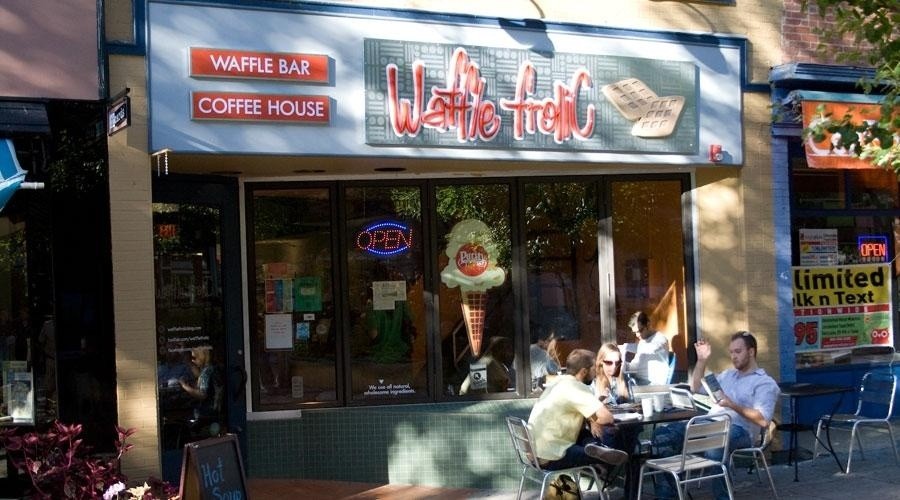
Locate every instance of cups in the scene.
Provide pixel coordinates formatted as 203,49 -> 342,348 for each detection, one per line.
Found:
652,393 -> 665,413
641,398 -> 653,418
708,144 -> 722,162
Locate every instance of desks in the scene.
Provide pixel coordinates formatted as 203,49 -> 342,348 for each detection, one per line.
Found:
747,382 -> 854,481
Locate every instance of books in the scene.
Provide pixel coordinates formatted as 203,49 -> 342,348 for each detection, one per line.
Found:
700,371 -> 724,404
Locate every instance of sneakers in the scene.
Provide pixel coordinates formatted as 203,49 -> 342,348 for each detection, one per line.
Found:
585,441 -> 628,466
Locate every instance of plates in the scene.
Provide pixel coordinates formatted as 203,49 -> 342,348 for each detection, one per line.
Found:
612,404 -> 637,410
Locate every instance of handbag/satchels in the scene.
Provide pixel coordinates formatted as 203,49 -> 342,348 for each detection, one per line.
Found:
545,474 -> 580,499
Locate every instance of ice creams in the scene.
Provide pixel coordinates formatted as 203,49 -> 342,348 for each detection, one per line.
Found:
438,219 -> 505,356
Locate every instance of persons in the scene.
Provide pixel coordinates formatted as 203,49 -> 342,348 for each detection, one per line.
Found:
590,342 -> 642,456
459,312 -> 670,395
652,332 -> 781,500
362,267 -> 417,364
179,347 -> 225,439
525,348 -> 629,478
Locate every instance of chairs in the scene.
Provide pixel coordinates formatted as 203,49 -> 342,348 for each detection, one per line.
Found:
180,369 -> 227,436
619,342 -> 636,375
668,351 -> 677,386
507,417 -> 610,499
636,414 -> 735,500
695,417 -> 781,499
808,372 -> 898,475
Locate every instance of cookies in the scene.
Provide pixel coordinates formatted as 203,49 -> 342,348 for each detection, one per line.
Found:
630,95 -> 684,136
602,77 -> 659,122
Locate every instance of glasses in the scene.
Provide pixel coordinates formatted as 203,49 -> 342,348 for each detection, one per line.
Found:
603,359 -> 621,366
